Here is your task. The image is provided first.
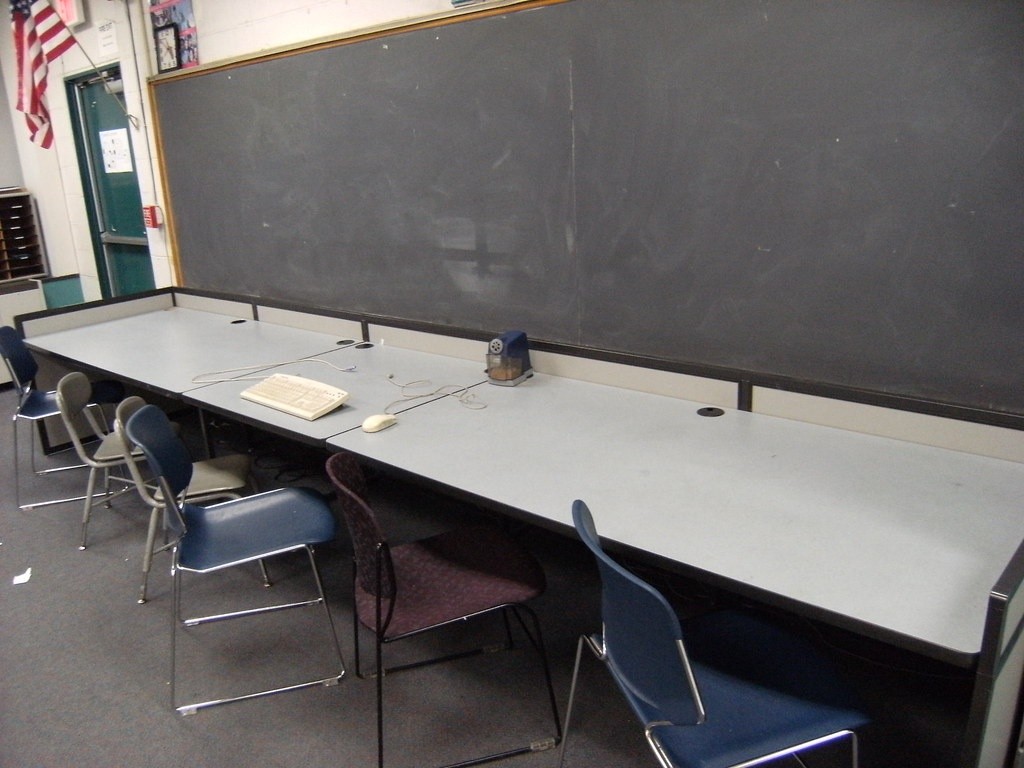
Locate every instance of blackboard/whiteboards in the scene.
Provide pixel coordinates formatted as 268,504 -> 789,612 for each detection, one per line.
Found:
146,3 -> 1023,434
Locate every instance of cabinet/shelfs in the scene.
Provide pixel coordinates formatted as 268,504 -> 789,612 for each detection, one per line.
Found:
0,279 -> 47,385
0,189 -> 51,285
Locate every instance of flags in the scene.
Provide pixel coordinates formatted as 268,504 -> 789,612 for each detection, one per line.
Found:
8,0 -> 76,149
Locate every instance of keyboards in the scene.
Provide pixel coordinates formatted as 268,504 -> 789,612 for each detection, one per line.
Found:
240,372 -> 348,421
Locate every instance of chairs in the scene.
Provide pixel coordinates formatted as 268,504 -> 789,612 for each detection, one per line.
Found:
0,326 -> 871,768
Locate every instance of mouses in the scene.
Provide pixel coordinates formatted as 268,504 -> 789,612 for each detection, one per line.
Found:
362,414 -> 398,433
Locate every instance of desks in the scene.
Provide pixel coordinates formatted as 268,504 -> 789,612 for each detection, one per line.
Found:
181,313 -> 500,450
14,286 -> 366,463
324,340 -> 1024,768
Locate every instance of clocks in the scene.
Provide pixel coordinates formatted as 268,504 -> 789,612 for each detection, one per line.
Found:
154,23 -> 181,75
